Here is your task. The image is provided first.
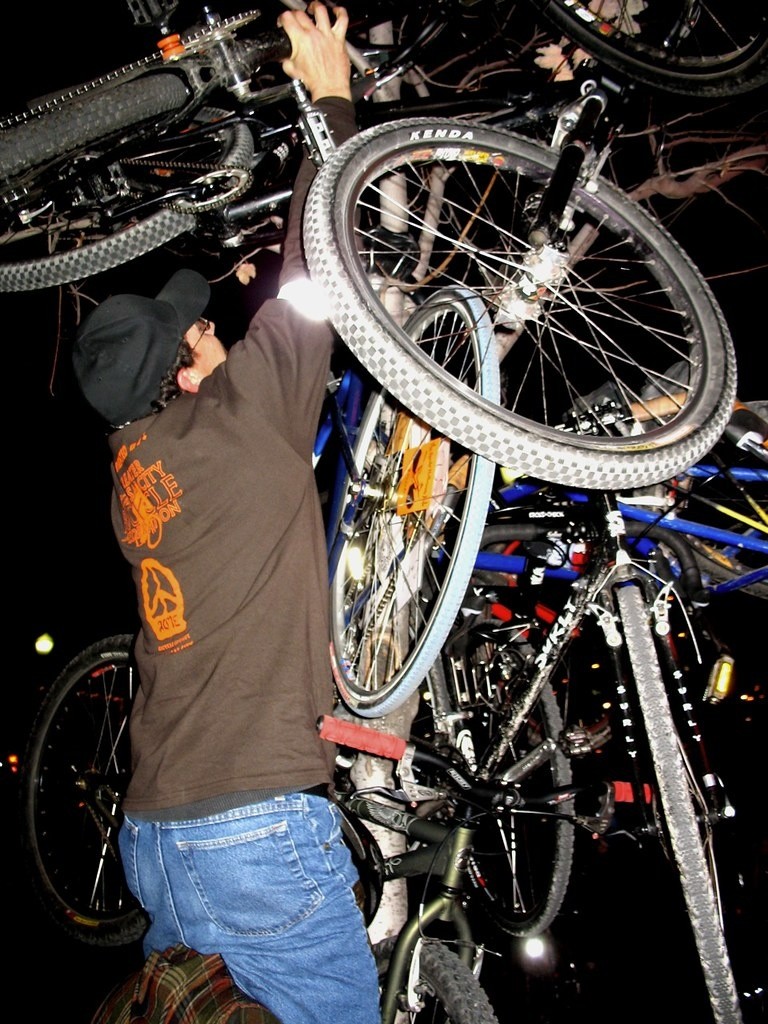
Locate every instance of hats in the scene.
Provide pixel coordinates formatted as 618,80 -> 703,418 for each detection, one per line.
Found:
72,270 -> 210,424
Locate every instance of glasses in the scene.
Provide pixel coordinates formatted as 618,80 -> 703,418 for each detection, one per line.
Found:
192,317 -> 211,352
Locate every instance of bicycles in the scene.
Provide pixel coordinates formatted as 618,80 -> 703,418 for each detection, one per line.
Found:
0,0 -> 768,1024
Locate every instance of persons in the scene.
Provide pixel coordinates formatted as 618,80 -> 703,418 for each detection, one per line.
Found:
71,0 -> 381,1024
